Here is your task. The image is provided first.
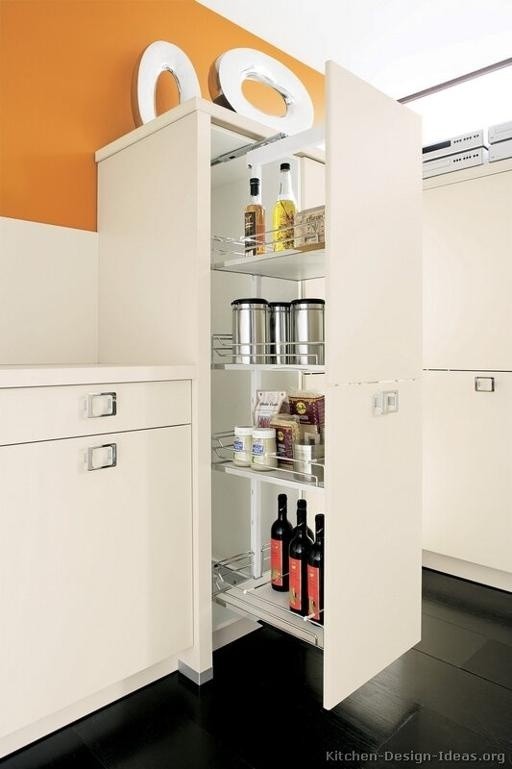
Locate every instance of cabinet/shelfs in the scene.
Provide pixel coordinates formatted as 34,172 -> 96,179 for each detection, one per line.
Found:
424,156 -> 512,627
97,59 -> 424,713
0,368 -> 195,763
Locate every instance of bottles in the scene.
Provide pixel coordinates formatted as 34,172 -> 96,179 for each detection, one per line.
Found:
268,493 -> 293,595
242,177 -> 266,254
306,513 -> 325,627
291,499 -> 314,539
273,163 -> 295,252
288,506 -> 313,617
229,296 -> 326,366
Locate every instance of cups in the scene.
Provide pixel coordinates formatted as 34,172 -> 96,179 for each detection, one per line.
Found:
291,440 -> 323,482
